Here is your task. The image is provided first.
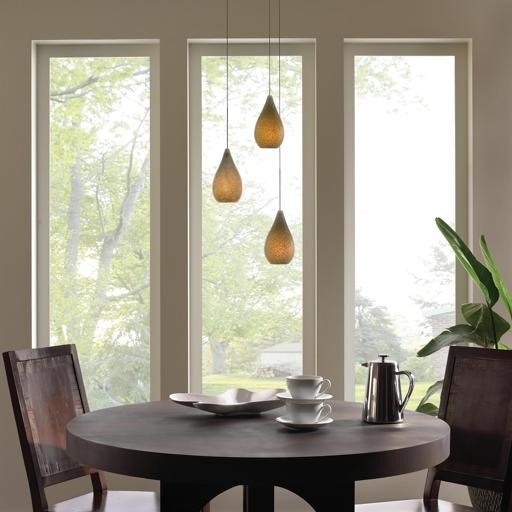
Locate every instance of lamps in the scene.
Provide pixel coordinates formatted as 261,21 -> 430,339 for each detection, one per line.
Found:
254,0 -> 284,149
263,0 -> 295,265
211,1 -> 243,202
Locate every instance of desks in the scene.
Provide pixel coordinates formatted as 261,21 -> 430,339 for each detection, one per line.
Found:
65,389 -> 451,511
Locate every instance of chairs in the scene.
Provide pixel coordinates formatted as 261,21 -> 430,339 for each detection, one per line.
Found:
2,343 -> 160,512
355,344 -> 512,512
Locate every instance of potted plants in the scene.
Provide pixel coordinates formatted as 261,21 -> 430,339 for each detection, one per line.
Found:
415,217 -> 512,512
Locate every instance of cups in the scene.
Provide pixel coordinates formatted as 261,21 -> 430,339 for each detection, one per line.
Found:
286,374 -> 331,398
284,402 -> 332,423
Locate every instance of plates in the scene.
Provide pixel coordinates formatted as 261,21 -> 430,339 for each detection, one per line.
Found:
276,415 -> 336,431
166,385 -> 290,417
276,390 -> 334,404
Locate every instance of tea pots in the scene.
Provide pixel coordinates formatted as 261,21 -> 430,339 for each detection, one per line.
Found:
360,353 -> 415,424
